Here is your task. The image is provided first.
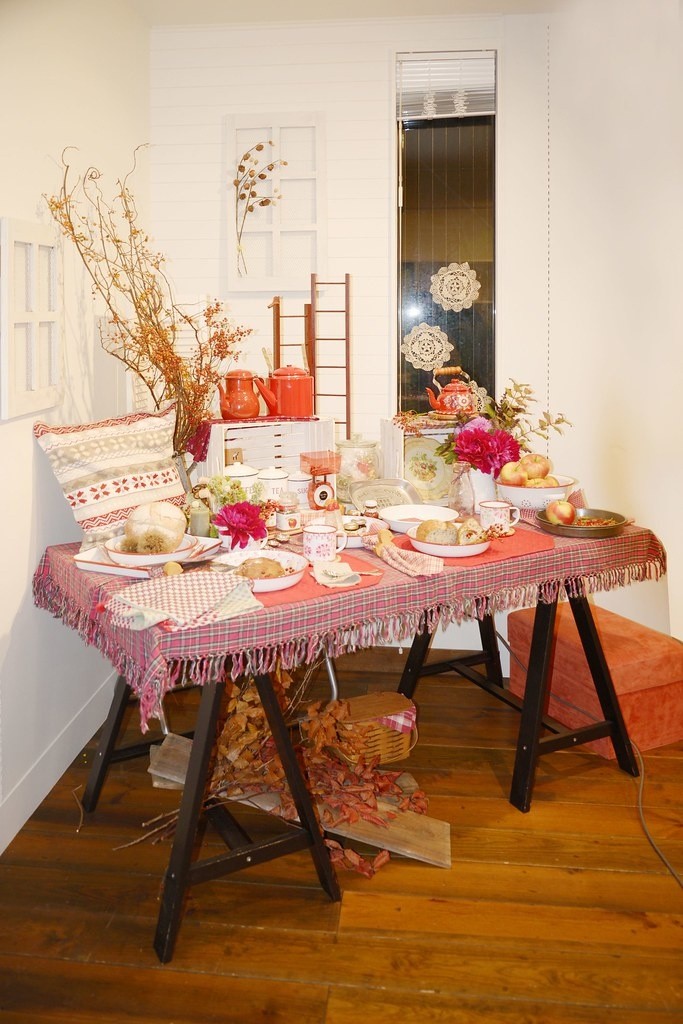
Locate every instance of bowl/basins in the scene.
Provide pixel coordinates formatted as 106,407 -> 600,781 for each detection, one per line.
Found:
406,522 -> 490,557
102,534 -> 198,568
207,549 -> 309,592
535,508 -> 628,538
380,504 -> 459,532
306,516 -> 390,548
492,473 -> 576,509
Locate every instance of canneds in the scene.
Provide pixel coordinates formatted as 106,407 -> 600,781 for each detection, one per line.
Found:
275,505 -> 301,534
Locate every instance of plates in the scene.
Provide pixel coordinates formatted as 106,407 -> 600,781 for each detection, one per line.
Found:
347,477 -> 423,512
403,435 -> 451,500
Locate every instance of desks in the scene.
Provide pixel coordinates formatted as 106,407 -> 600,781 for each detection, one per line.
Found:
34,504 -> 667,962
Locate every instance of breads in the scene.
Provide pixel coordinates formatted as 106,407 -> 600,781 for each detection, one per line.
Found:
415,518 -> 486,546
233,556 -> 285,579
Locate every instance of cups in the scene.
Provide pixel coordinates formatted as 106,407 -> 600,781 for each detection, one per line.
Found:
287,471 -> 313,504
479,500 -> 521,532
302,525 -> 347,560
257,466 -> 288,501
225,462 -> 259,501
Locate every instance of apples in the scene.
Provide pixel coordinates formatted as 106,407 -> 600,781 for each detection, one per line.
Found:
546,501 -> 576,526
499,453 -> 560,487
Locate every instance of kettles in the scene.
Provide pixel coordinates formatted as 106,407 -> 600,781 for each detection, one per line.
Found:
425,365 -> 475,415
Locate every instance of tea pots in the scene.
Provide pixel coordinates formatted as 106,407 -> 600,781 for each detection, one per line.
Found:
214,369 -> 264,420
254,364 -> 315,417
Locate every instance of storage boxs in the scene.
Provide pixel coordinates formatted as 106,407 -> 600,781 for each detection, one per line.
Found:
380,416 -> 498,507
507,598 -> 683,761
207,419 -> 338,500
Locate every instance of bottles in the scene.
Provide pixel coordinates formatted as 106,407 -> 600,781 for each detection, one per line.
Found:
335,432 -> 383,502
363,500 -> 378,519
346,509 -> 367,536
446,461 -> 475,520
276,493 -> 301,531
333,501 -> 344,529
325,504 -> 337,529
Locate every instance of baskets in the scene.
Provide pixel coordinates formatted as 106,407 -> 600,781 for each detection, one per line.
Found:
331,692 -> 418,768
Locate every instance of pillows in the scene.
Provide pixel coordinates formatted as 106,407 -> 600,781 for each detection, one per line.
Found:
32,402 -> 197,554
